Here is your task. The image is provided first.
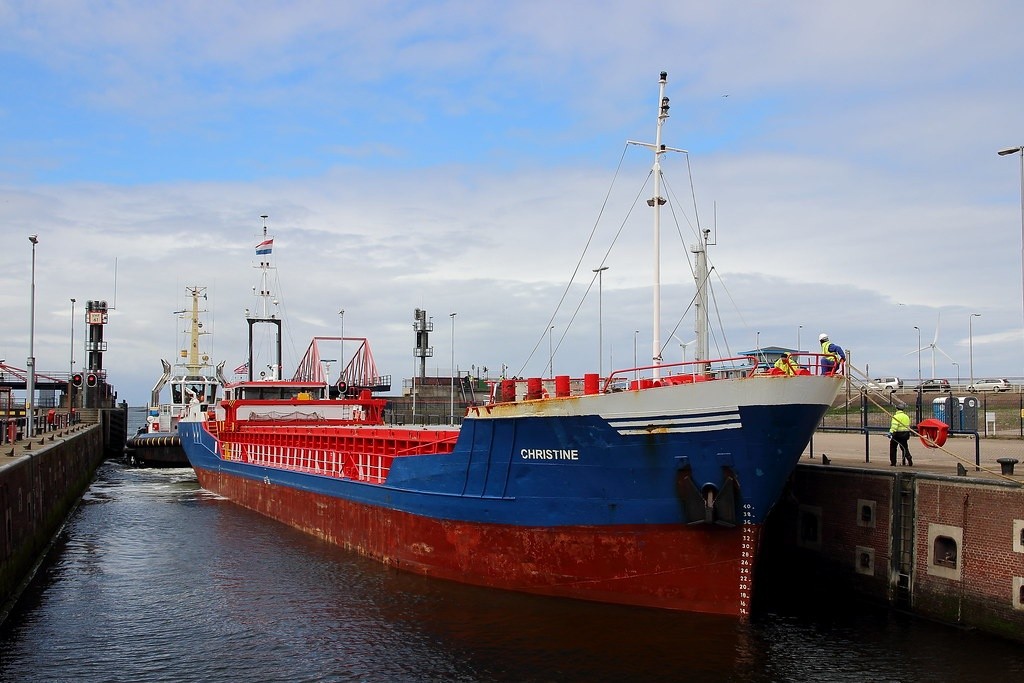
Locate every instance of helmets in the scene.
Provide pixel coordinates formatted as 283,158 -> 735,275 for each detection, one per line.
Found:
819,334 -> 828,341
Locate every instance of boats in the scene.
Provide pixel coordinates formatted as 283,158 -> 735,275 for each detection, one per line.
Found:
167,68 -> 857,621
124,284 -> 232,468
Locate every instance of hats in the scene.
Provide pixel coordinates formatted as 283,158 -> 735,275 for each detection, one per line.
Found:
896,404 -> 904,410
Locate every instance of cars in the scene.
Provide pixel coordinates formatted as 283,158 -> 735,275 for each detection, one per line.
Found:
965,378 -> 1012,393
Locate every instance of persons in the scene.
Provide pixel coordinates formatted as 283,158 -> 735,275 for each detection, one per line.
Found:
773,351 -> 807,375
172,385 -> 204,404
465,375 -> 518,387
10,392 -> 15,408
819,333 -> 846,375
888,404 -> 913,467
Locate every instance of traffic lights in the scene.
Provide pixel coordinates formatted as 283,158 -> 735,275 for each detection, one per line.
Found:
72,374 -> 83,387
338,382 -> 348,393
86,374 -> 97,387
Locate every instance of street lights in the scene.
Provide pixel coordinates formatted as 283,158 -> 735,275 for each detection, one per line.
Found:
797,324 -> 804,364
70,297 -> 76,381
914,325 -> 922,387
969,312 -> 982,393
548,325 -> 555,380
951,363 -> 960,385
21,234 -> 40,437
634,329 -> 640,380
449,311 -> 457,427
994,143 -> 1024,278
755,331 -> 761,369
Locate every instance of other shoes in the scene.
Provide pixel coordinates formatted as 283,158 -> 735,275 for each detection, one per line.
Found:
891,463 -> 896,466
908,460 -> 913,466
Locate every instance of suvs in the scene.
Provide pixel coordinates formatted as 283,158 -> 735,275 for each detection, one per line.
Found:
913,378 -> 951,393
860,376 -> 904,393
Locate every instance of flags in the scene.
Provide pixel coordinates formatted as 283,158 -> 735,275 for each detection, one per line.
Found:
256,239 -> 273,255
234,362 -> 250,374
